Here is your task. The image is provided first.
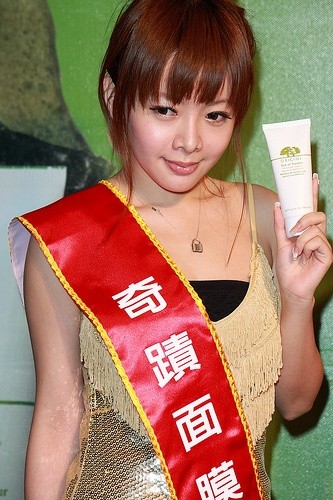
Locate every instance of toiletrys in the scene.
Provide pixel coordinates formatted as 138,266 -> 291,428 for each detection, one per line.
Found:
262,118 -> 313,238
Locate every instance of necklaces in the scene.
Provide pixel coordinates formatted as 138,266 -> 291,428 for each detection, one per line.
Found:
130,181 -> 204,254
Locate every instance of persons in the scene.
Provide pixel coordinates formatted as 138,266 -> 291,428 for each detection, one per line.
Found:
8,0 -> 333,500
